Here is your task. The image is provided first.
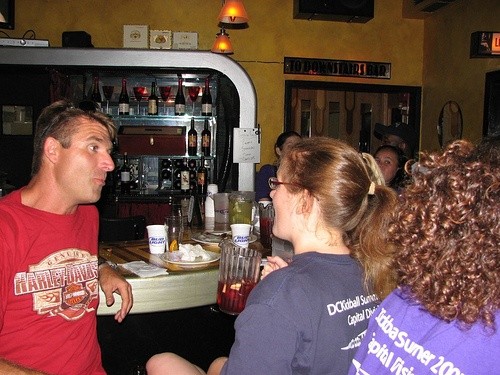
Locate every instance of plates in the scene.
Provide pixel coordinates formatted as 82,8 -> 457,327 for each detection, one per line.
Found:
160,250 -> 222,265
192,232 -> 257,245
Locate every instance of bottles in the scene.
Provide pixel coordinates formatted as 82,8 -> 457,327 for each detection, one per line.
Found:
201,77 -> 213,116
174,78 -> 186,117
179,158 -> 190,195
118,78 -> 130,116
188,119 -> 197,156
147,82 -> 159,115
121,152 -> 130,191
87,77 -> 103,114
201,119 -> 212,157
197,156 -> 207,196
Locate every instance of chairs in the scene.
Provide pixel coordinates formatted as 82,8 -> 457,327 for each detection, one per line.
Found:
99,215 -> 146,243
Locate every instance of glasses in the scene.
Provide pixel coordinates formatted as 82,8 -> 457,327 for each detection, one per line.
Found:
267,175 -> 321,202
39,99 -> 99,137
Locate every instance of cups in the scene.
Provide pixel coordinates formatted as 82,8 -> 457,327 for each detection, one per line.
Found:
217,244 -> 270,316
205,184 -> 274,247
145,216 -> 184,254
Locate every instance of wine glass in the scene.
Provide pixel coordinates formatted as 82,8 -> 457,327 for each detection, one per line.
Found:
159,86 -> 172,115
133,86 -> 145,115
188,86 -> 200,116
101,85 -> 114,113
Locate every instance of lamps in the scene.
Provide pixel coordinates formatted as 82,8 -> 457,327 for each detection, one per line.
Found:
209,0 -> 249,54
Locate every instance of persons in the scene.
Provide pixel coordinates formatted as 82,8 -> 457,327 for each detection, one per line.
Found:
147,136 -> 398,375
0,98 -> 133,375
375,120 -> 414,160
375,145 -> 406,187
347,140 -> 500,375
256,131 -> 301,203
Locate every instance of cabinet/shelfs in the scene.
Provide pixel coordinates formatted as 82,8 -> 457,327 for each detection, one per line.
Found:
56,68 -> 220,204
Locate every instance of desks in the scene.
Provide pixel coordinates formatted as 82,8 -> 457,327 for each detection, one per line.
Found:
97,228 -> 263,314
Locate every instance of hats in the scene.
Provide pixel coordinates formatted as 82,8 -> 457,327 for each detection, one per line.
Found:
374,120 -> 414,145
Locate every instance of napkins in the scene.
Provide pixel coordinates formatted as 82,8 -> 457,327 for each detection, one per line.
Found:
180,243 -> 208,261
117,260 -> 170,279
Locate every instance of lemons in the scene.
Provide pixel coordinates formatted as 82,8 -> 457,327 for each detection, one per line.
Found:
170,240 -> 178,252
